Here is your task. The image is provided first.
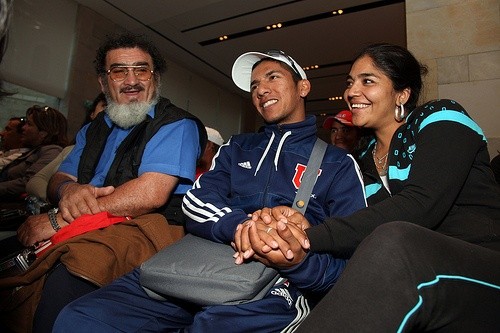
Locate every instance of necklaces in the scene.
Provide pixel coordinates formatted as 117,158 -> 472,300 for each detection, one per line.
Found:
373,141 -> 390,176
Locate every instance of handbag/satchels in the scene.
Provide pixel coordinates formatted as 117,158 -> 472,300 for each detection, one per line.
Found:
141,233 -> 286,305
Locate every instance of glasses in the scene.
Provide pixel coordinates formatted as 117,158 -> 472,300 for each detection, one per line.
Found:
103,66 -> 155,82
44,105 -> 50,116
260,49 -> 300,76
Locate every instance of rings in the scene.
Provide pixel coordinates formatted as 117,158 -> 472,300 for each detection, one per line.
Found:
266,227 -> 273,234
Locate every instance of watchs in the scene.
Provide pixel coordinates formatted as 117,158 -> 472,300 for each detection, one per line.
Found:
47,207 -> 61,232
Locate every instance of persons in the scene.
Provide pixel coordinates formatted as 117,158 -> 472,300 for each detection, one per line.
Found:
0,32 -> 209,333
0,91 -> 108,262
0,116 -> 32,173
51,44 -> 500,333
0,105 -> 67,205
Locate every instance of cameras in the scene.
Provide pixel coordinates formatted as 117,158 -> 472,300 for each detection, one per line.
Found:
0,247 -> 36,278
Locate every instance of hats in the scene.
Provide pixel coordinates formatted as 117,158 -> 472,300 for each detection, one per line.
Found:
205,126 -> 224,146
322,109 -> 354,130
231,50 -> 307,93
